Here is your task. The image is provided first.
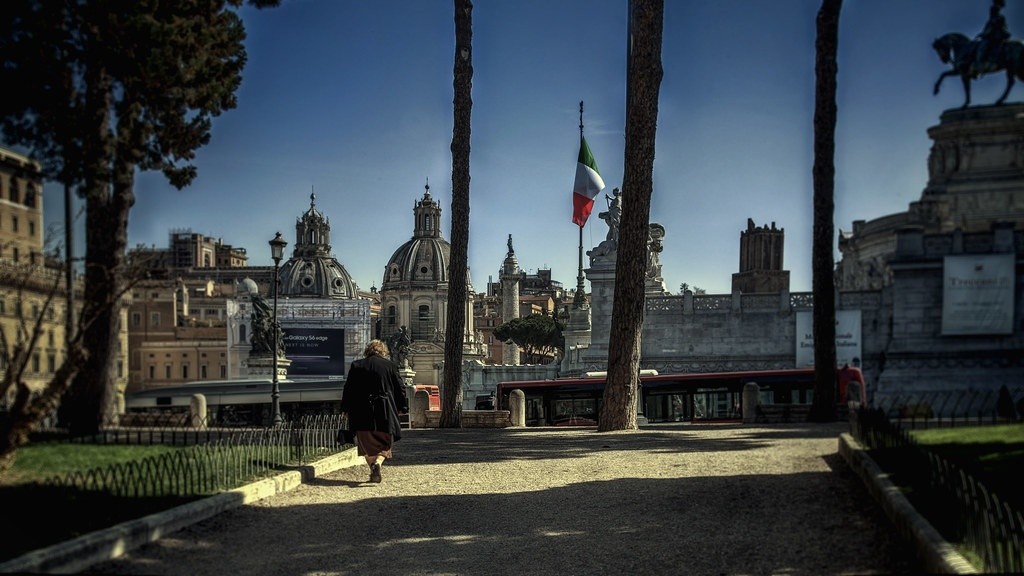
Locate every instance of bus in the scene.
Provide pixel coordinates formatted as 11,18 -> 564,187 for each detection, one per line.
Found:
497,363 -> 866,427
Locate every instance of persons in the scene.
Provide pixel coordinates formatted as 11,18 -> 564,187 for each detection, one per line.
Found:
248,293 -> 286,359
970,0 -> 1011,79
586,186 -> 622,267
340,339 -> 408,484
645,224 -> 660,281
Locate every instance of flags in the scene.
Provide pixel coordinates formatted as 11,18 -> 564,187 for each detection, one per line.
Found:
572,133 -> 605,228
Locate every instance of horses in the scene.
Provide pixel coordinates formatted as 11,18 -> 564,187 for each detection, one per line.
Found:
931,33 -> 1024,109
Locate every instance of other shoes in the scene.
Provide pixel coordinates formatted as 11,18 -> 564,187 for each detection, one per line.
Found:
370,464 -> 376,482
374,463 -> 381,483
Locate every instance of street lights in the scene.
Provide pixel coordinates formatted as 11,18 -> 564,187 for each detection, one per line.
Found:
269,232 -> 288,428
